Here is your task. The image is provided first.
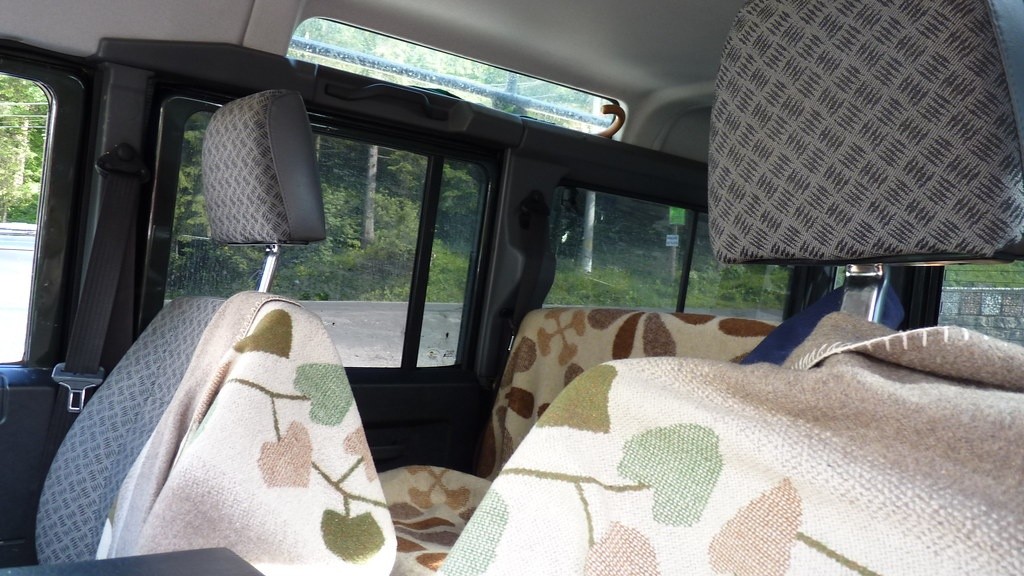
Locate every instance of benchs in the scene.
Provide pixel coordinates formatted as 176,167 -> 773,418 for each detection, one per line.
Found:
379,307 -> 783,576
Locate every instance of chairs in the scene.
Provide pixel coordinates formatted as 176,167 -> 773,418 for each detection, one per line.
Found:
34,85 -> 398,576
429,0 -> 1024,576
